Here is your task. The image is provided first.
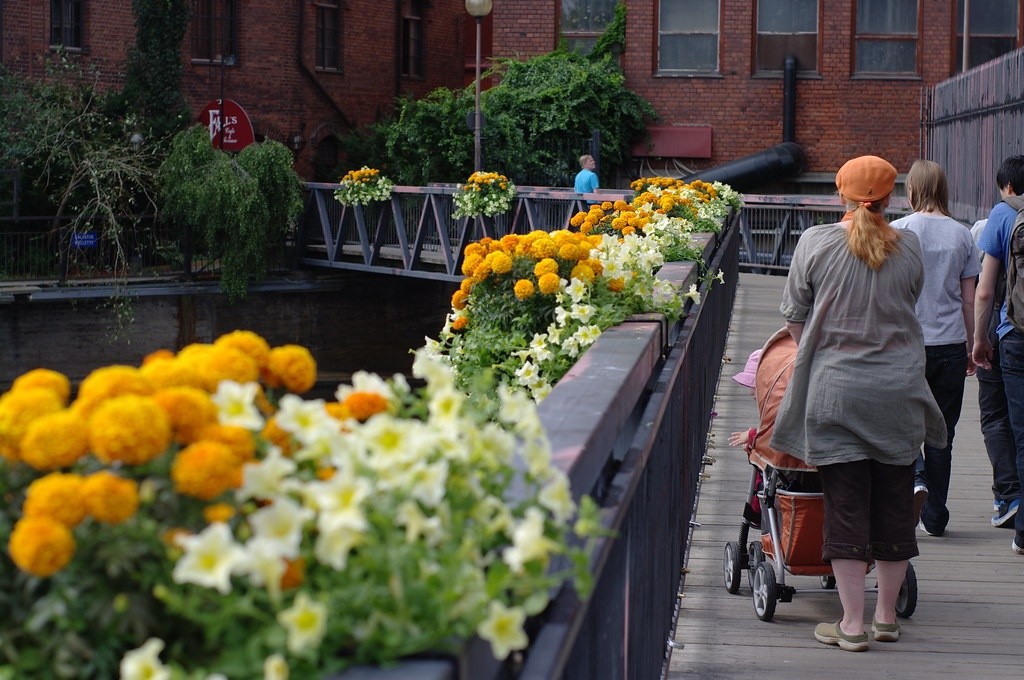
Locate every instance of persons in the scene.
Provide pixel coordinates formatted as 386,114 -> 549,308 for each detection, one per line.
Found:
972,155 -> 1024,553
727,349 -> 763,447
889,160 -> 982,536
574,155 -> 600,203
778,157 -> 948,652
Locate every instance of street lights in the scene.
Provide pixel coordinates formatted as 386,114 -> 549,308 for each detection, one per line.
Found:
460,0 -> 497,171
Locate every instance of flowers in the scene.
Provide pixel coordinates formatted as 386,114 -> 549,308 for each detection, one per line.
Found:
0,331 -> 621,680
451,170 -> 518,220
333,165 -> 394,207
410,229 -> 703,431
569,199 -> 725,290
629,176 -> 746,238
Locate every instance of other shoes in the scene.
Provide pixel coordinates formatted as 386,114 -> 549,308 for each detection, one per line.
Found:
913,483 -> 928,528
918,516 -> 937,536
994,499 -> 1002,512
1011,533 -> 1024,553
991,498 -> 1022,527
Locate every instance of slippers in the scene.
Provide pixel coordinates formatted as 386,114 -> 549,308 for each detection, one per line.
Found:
814,617 -> 869,651
870,612 -> 901,641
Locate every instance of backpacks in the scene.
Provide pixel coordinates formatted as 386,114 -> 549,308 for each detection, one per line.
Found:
1001,196 -> 1024,333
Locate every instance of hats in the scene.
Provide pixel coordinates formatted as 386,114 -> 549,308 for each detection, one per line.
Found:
731,349 -> 763,388
835,155 -> 898,202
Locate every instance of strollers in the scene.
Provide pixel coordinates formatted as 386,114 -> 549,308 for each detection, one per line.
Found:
721,323 -> 919,623
